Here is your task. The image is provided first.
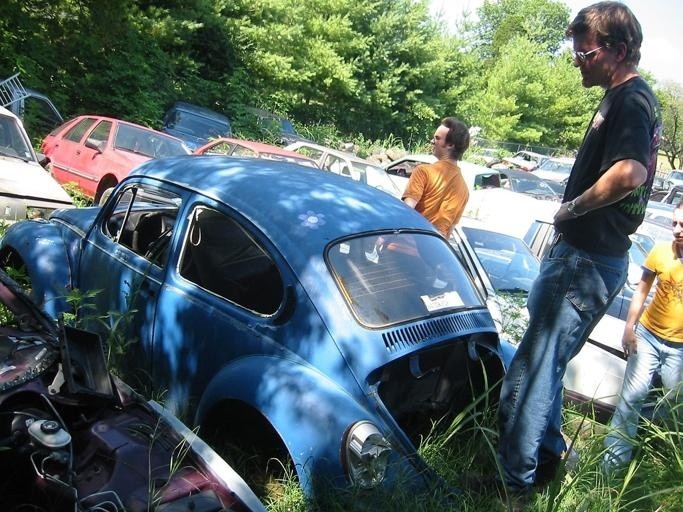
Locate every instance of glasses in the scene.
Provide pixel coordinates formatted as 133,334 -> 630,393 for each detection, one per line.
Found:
570,46 -> 605,63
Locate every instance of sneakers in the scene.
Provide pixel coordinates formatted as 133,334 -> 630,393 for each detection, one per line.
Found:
460,471 -> 527,511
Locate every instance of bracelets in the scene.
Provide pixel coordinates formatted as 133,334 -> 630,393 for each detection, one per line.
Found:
567,196 -> 587,217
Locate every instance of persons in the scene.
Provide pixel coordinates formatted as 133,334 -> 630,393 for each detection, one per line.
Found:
378,115 -> 470,282
458,0 -> 662,502
593,201 -> 683,479
